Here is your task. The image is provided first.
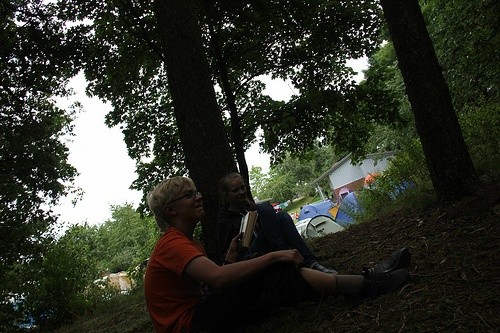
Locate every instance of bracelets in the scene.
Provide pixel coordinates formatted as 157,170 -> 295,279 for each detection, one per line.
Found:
223,260 -> 232,265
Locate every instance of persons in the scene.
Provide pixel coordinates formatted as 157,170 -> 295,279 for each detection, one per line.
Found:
324,189 -> 329,200
142,177 -> 412,333
295,211 -> 299,219
215,174 -> 339,291
330,190 -> 334,199
299,205 -> 303,210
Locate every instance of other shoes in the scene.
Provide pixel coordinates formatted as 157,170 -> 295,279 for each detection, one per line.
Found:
310,262 -> 338,276
346,269 -> 410,301
368,246 -> 412,274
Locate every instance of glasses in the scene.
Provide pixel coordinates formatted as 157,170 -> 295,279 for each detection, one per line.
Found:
166,192 -> 201,204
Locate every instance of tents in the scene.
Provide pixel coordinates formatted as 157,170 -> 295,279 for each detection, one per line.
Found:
94,271 -> 137,296
295,173 -> 384,240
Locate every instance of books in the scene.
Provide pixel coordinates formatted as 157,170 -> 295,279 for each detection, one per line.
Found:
238,210 -> 258,248
